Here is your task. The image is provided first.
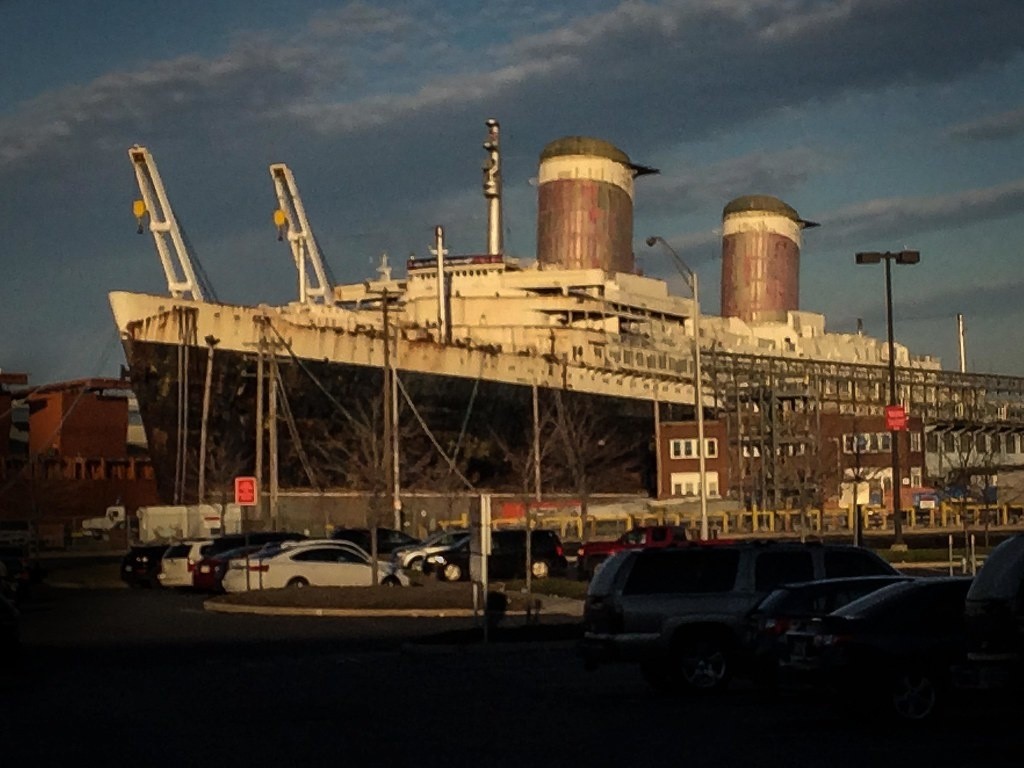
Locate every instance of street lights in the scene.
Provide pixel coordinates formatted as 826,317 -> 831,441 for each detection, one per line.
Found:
646,234 -> 711,540
857,251 -> 920,552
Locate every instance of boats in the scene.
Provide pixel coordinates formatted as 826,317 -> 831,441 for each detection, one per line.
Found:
107,117 -> 967,495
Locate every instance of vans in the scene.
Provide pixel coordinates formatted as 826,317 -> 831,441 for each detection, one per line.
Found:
585,545 -> 902,692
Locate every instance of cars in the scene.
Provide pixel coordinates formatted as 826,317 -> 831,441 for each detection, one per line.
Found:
0,519 -> 32,635
740,536 -> 1024,721
118,526 -> 736,592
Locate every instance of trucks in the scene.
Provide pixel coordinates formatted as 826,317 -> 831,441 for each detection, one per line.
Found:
82,505 -> 246,540
